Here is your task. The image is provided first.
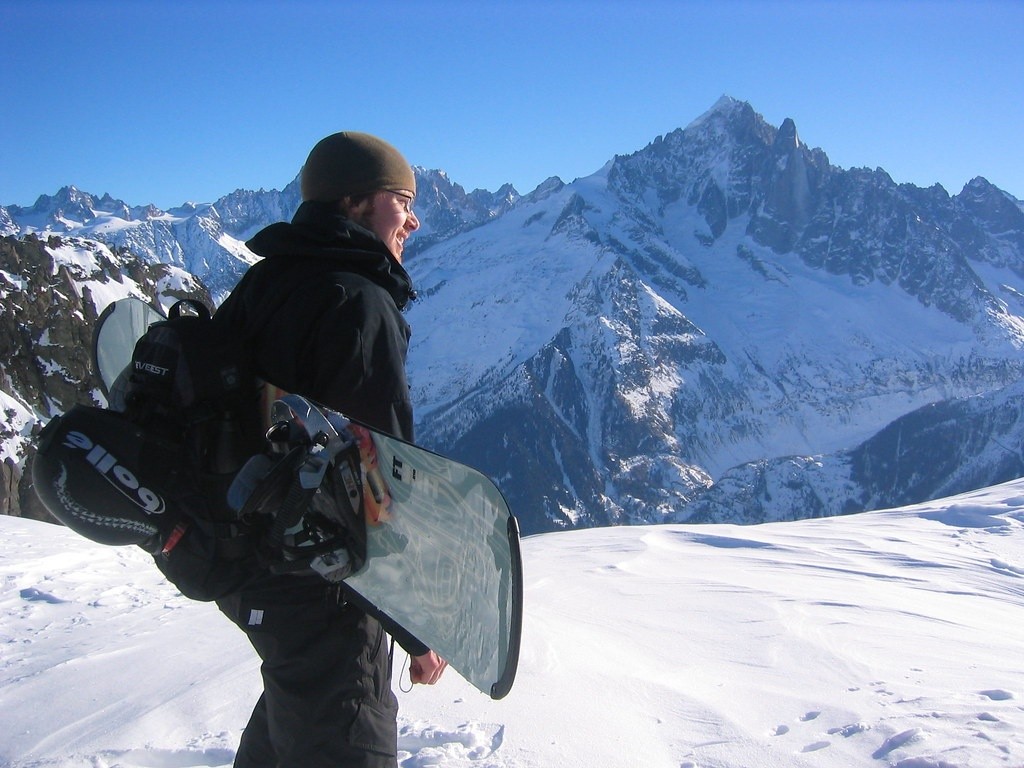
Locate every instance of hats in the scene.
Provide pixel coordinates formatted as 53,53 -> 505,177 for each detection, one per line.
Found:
300,131 -> 417,203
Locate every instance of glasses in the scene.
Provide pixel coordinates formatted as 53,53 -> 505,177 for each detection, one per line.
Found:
387,190 -> 416,213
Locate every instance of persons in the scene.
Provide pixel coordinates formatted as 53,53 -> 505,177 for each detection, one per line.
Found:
211,132 -> 450,768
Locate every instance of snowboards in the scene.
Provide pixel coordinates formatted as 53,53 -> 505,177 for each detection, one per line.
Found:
88,295 -> 524,701
